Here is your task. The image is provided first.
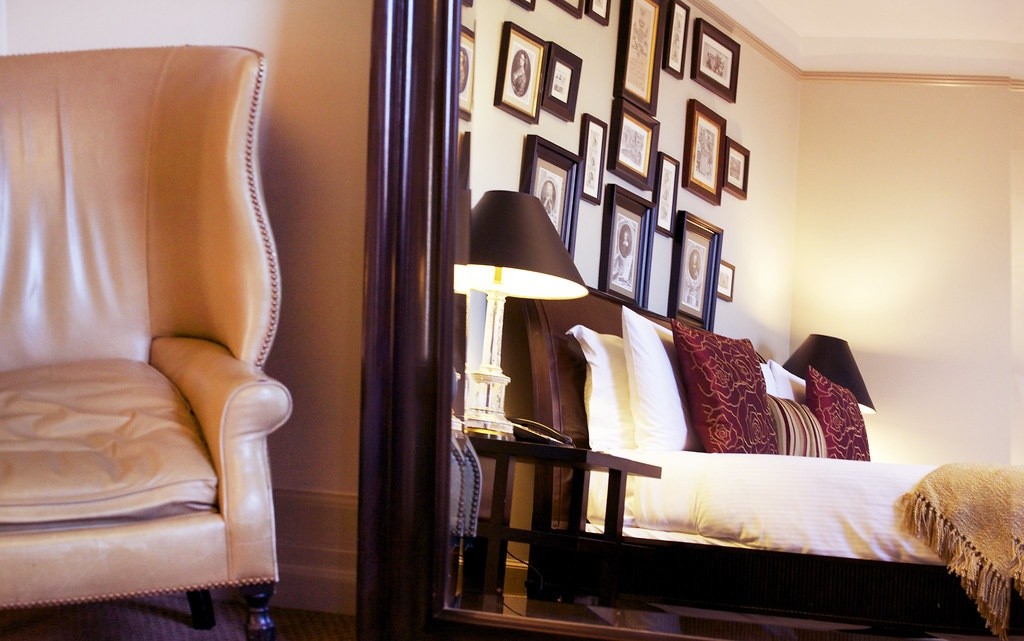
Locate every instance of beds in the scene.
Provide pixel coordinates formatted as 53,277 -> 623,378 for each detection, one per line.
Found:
517,285 -> 1021,636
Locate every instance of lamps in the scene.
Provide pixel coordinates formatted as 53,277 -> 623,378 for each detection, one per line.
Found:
466,189 -> 595,438
782,335 -> 877,415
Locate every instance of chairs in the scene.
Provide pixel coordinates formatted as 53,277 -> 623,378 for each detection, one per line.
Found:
0,47 -> 298,641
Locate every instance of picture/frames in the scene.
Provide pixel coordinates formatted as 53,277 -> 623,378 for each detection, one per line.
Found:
460,0 -> 751,332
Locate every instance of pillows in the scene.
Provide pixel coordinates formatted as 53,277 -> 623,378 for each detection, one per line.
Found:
759,395 -> 831,461
768,362 -> 807,427
666,322 -> 780,457
804,366 -> 876,461
621,306 -> 778,454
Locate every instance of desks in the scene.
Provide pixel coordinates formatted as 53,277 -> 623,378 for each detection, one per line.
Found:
468,438 -> 661,608
